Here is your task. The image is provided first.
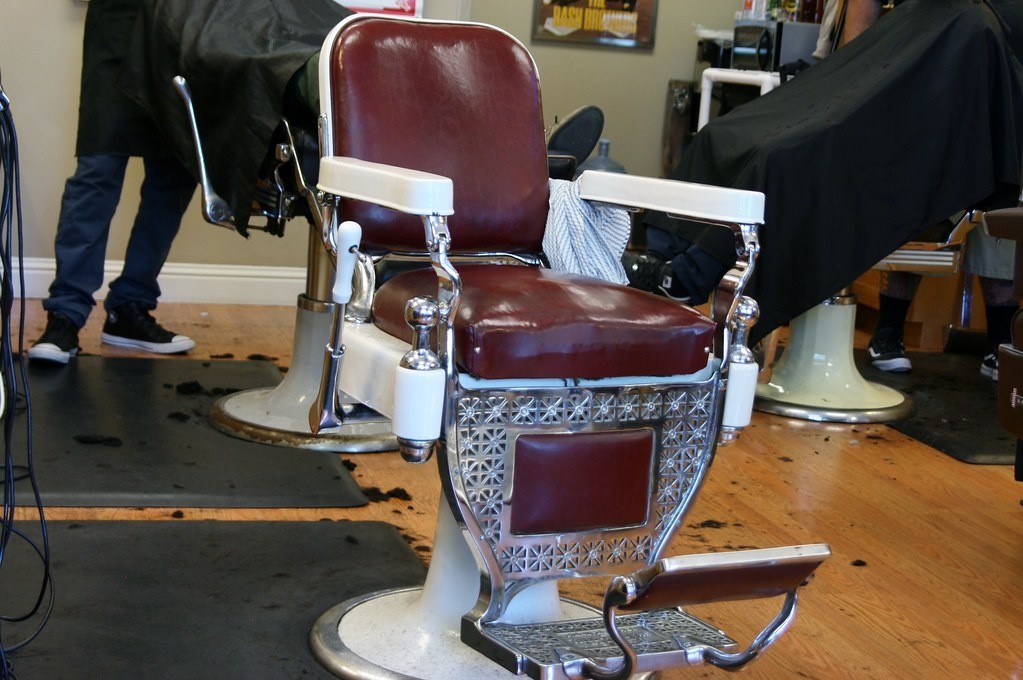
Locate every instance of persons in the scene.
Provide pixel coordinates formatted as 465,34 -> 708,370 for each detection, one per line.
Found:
119,2 -> 605,169
619,0 -> 1021,347
26,1 -> 197,365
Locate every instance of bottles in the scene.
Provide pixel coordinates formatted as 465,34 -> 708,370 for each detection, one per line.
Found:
765,0 -> 781,22
783,0 -> 802,22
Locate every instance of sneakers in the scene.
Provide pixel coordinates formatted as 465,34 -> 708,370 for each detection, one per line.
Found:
621,250 -> 691,302
28,314 -> 80,364
979,348 -> 1020,381
866,327 -> 918,374
101,308 -> 195,355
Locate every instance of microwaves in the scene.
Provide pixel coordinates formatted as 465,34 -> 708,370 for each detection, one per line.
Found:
730,18 -> 820,72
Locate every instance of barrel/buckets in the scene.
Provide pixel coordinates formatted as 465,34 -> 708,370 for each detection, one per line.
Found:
575,139 -> 627,182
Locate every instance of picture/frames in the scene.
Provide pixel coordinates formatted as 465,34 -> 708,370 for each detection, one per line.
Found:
530,0 -> 661,51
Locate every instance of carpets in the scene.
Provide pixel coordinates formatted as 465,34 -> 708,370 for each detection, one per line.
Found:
0,350 -> 368,511
2,521 -> 430,679
755,348 -> 1022,466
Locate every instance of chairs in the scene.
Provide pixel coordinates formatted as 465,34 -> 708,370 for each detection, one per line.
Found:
173,69 -> 403,455
751,206 -> 985,426
313,9 -> 833,678
